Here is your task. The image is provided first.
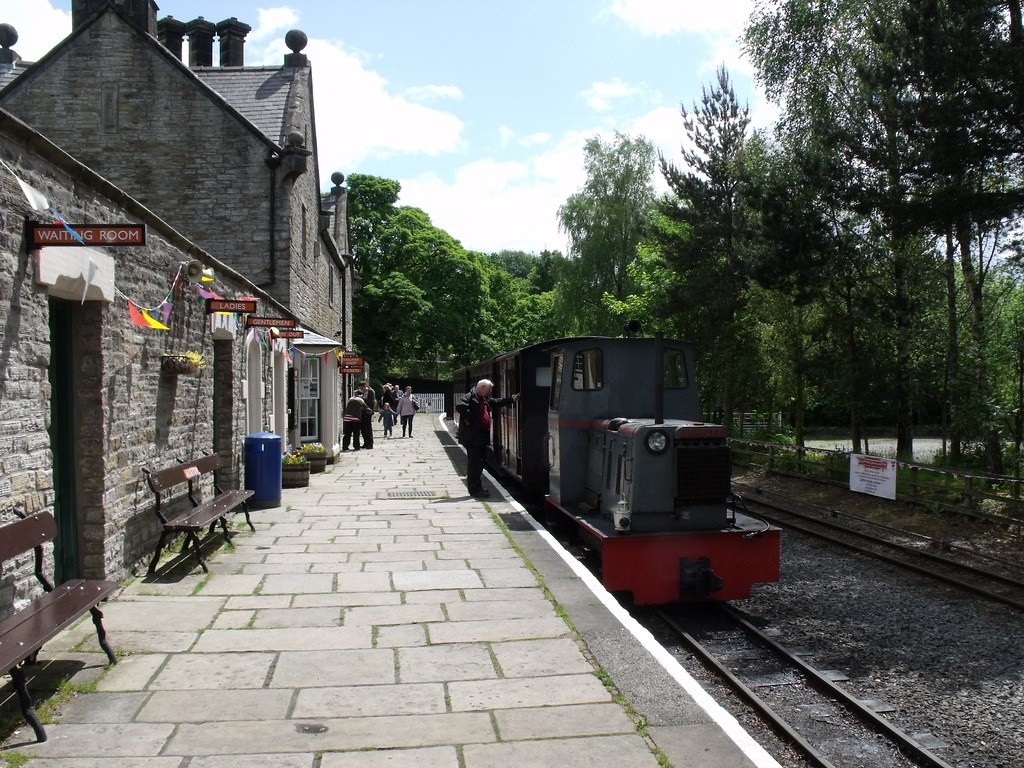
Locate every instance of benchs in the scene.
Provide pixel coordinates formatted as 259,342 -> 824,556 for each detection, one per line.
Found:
141,451 -> 254,576
0,511 -> 119,742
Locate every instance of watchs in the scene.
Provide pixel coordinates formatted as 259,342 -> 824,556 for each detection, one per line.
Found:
414,401 -> 416,402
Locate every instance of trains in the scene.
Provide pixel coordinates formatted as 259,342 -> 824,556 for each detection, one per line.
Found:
451,318 -> 783,610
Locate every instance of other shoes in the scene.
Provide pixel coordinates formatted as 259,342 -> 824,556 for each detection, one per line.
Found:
409,435 -> 413,438
355,445 -> 359,450
403,431 -> 405,437
471,489 -> 489,497
360,444 -> 365,447
343,447 -> 349,452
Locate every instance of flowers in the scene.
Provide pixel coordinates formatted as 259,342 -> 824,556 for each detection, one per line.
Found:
293,442 -> 325,453
281,450 -> 308,465
164,350 -> 206,374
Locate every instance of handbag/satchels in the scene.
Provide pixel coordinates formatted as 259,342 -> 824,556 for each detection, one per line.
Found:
412,395 -> 419,410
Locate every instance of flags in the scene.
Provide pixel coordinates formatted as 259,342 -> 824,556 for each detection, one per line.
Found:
126,273 -> 180,336
196,285 -> 344,367
12,175 -> 97,305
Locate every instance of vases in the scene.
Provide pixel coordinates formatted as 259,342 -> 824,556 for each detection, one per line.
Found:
282,461 -> 311,488
294,451 -> 328,474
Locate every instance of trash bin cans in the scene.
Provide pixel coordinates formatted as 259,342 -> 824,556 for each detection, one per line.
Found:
244,433 -> 282,507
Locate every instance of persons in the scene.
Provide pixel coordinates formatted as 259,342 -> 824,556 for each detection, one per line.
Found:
455,379 -> 520,498
358,380 -> 375,449
379,383 -> 403,425
397,386 -> 419,437
342,390 -> 371,452
378,402 -> 397,438
